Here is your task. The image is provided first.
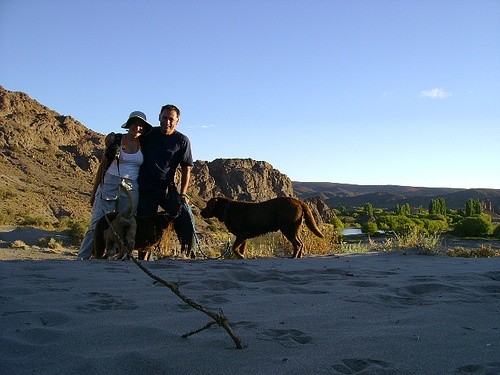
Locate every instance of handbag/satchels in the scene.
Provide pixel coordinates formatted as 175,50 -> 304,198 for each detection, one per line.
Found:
101,186 -> 120,201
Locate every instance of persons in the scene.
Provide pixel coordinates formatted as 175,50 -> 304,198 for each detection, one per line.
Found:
104,104 -> 197,262
76,111 -> 153,260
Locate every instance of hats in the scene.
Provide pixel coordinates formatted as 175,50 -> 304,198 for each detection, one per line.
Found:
121,111 -> 154,135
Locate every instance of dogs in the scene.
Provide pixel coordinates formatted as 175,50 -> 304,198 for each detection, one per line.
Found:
201,197 -> 325,259
